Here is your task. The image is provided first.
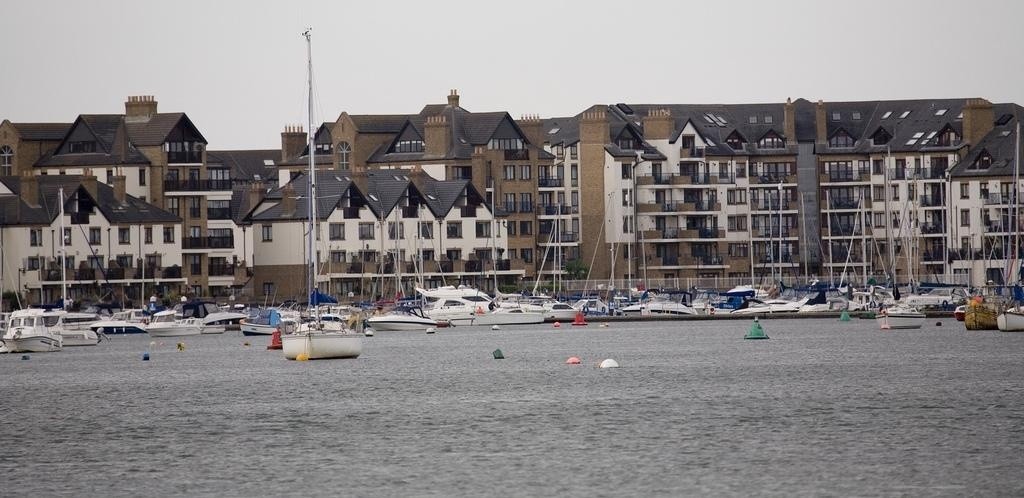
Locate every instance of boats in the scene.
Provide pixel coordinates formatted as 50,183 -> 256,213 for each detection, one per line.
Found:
0,154 -> 1024,353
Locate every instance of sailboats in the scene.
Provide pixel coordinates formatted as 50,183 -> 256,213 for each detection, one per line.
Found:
279,28 -> 365,361
874,147 -> 926,329
